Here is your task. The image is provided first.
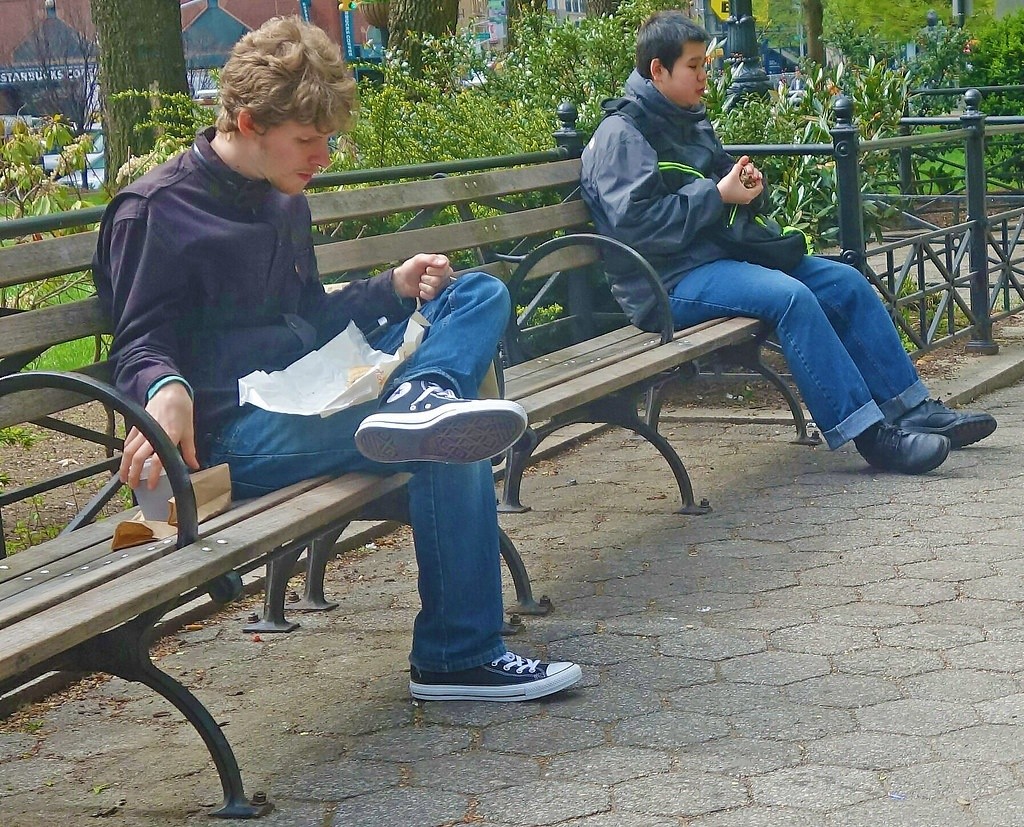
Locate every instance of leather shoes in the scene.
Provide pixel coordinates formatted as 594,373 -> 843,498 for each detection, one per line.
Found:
854,421 -> 951,474
894,396 -> 997,448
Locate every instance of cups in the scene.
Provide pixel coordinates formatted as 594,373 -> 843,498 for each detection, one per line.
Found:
128,454 -> 175,522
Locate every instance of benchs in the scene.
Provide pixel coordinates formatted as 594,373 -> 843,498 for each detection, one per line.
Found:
1,157 -> 823,819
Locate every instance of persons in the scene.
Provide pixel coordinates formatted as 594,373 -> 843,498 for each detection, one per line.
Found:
90,15 -> 581,702
582,12 -> 997,473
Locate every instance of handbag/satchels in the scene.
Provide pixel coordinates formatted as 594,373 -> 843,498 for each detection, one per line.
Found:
660,159 -> 808,269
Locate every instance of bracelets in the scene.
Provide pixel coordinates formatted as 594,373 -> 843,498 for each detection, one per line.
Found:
147,376 -> 194,400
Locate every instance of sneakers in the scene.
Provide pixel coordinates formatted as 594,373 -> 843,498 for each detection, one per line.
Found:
353,380 -> 528,466
409,652 -> 581,701
